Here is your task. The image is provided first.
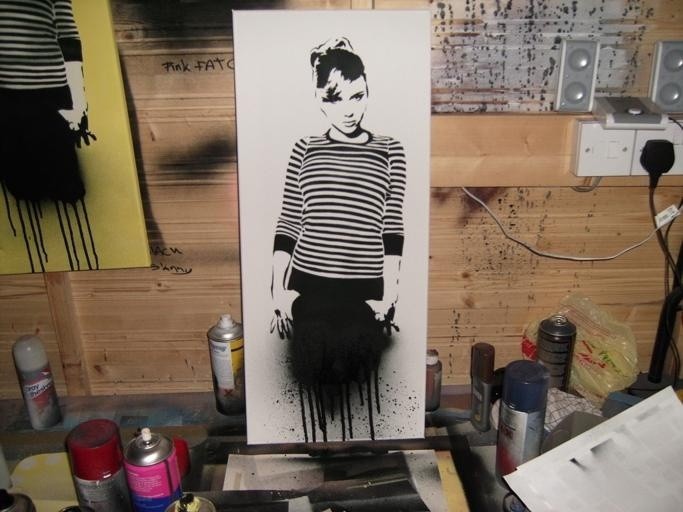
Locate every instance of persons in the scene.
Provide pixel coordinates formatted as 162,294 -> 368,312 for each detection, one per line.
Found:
270,36 -> 407,386
0,0 -> 87,203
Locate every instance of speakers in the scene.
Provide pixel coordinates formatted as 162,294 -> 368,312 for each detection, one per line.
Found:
555,39 -> 601,113
649,41 -> 683,112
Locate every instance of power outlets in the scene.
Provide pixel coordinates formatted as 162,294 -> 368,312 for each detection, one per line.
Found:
631,120 -> 683,176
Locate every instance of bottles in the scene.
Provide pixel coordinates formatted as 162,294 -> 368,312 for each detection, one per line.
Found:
425,349 -> 442,412
